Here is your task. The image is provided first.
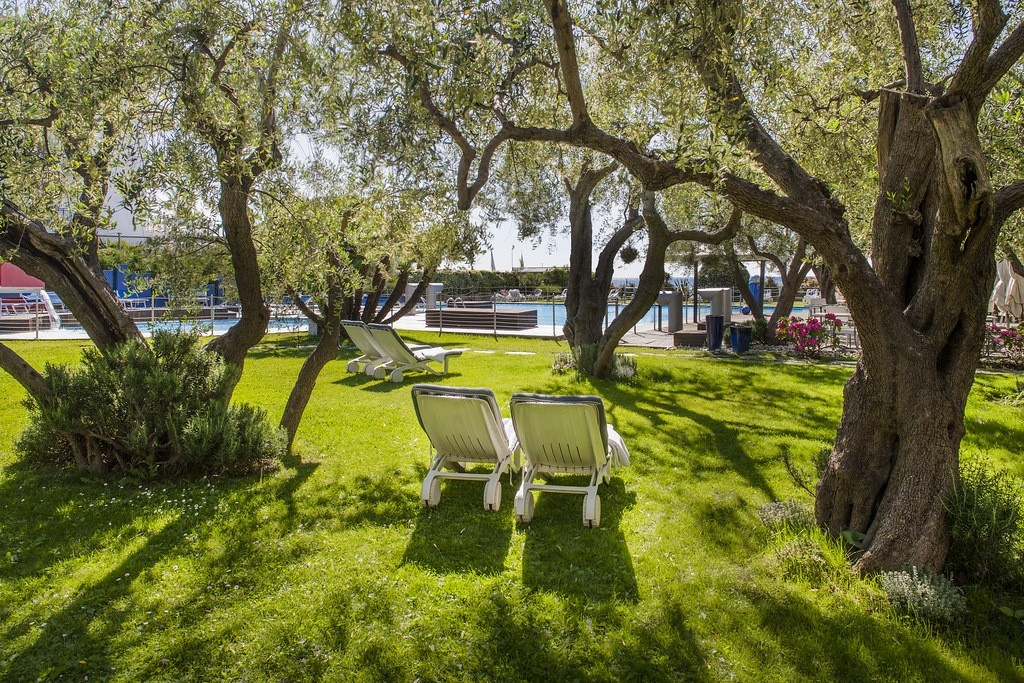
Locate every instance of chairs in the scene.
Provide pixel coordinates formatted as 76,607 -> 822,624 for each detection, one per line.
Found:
494,289 -> 568,302
510,392 -> 613,527
411,384 -> 521,512
341,320 -> 432,376
368,323 -> 462,382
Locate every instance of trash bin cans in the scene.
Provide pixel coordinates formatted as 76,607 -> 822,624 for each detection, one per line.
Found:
729,324 -> 753,354
705,315 -> 724,351
